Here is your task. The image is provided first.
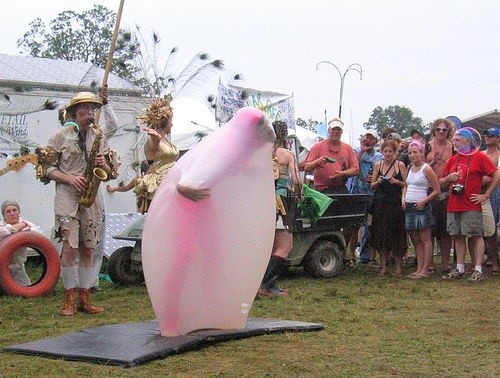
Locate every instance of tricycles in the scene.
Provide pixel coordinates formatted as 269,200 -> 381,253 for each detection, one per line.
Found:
105,133 -> 374,290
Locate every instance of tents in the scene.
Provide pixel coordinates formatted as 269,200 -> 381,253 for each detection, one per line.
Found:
0,54 -> 144,254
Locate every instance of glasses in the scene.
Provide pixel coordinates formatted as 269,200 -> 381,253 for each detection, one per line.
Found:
435,128 -> 447,132
486,133 -> 494,138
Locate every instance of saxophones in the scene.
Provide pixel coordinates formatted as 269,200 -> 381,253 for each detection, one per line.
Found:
79,118 -> 108,208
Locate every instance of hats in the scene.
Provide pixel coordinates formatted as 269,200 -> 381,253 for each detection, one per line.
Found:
329,120 -> 344,130
1,199 -> 20,215
485,127 -> 500,136
66,92 -> 103,112
410,128 -> 425,138
387,133 -> 401,141
364,128 -> 378,138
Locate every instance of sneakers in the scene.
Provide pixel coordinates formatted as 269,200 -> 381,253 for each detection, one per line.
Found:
467,270 -> 485,282
442,269 -> 465,280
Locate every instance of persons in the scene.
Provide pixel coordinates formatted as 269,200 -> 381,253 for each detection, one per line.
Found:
346,129 -> 384,264
59,83 -> 118,293
401,141 -> 440,279
371,140 -> 408,275
258,121 -> 302,297
0,199 -> 36,286
107,159 -> 154,192
382,128 -> 500,270
36,92 -> 122,316
438,129 -> 500,281
133,99 -> 179,215
424,120 -> 457,274
302,118 -> 359,191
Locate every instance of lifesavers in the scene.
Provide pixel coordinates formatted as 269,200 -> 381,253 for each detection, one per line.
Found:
0,231 -> 61,298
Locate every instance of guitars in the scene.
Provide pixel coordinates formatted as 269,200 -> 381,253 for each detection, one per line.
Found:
0,154 -> 37,176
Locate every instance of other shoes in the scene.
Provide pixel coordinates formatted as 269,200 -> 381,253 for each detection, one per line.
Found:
491,270 -> 499,275
406,272 -> 430,280
257,287 -> 289,298
60,289 -> 77,315
78,288 -> 104,314
397,272 -> 403,277
368,260 -> 381,269
349,258 -> 356,268
376,270 -> 389,276
441,265 -> 452,272
428,268 -> 436,274
